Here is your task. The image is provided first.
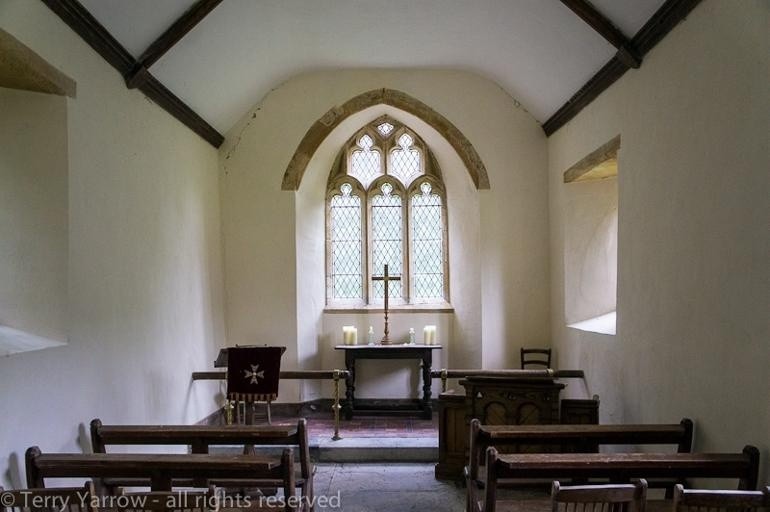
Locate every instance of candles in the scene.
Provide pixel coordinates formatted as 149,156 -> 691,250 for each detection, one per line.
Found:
424,325 -> 437,344
342,325 -> 358,345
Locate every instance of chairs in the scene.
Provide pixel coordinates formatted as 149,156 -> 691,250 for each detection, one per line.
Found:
520,346 -> 552,369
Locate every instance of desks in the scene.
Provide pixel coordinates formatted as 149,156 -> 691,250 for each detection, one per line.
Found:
435,372 -> 600,492
334,344 -> 443,421
